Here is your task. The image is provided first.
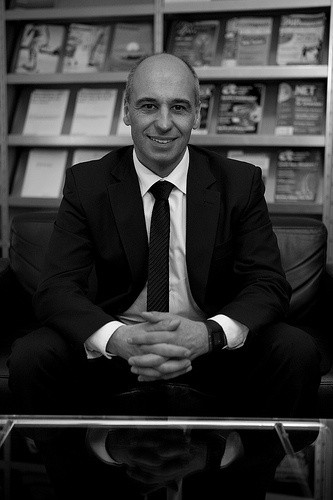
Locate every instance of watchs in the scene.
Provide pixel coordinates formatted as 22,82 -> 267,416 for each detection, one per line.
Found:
203,320 -> 225,353
204,434 -> 227,472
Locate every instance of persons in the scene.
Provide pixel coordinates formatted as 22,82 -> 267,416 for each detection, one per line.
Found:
12,426 -> 319,500
8,55 -> 322,500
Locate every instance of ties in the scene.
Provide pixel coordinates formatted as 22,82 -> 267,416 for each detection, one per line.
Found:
147,180 -> 175,311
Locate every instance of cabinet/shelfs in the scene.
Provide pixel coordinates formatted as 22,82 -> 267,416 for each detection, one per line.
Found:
1,0 -> 333,262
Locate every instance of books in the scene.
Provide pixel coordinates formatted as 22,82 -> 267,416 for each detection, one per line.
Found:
22,88 -> 132,138
227,150 -> 320,204
168,13 -> 325,68
194,84 -> 325,138
72,150 -> 108,168
19,150 -> 67,200
16,24 -> 152,77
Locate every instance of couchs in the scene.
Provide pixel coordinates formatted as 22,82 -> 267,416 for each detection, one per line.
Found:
3,213 -> 333,399
